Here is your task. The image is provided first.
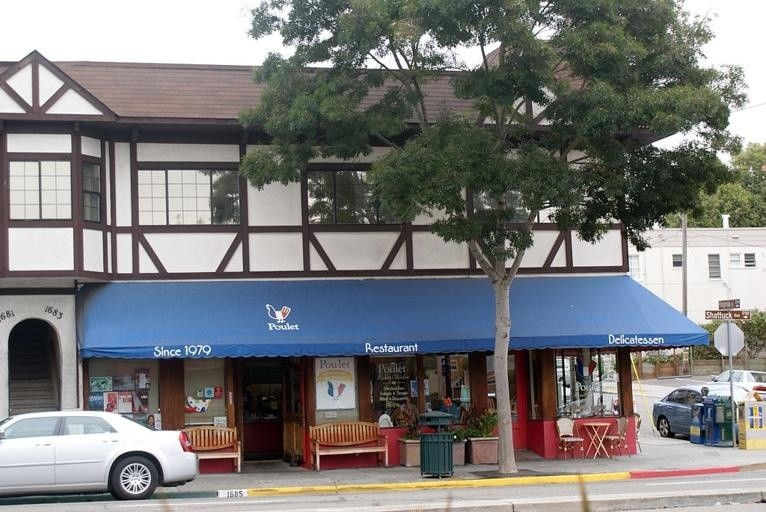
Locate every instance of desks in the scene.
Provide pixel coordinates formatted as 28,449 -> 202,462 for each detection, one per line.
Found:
581,421 -> 613,459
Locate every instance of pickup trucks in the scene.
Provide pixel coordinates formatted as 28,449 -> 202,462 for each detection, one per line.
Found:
454,384 -> 470,408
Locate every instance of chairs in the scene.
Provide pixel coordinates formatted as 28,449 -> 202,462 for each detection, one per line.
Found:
600,416 -> 632,458
555,417 -> 585,462
632,412 -> 642,454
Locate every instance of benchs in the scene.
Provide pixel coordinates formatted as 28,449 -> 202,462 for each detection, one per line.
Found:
307,421 -> 389,472
173,425 -> 243,474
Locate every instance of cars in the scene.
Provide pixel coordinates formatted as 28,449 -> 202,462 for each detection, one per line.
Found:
0,408 -> 198,500
653,381 -> 751,441
556,358 -> 570,387
709,369 -> 766,401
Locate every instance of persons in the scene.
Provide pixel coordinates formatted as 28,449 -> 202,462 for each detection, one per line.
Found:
147,414 -> 156,428
391,395 -> 419,426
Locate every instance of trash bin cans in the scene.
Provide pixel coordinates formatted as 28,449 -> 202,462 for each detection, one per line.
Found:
417,411 -> 456,479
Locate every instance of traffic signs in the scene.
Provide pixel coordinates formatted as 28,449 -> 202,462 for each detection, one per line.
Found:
706,311 -> 750,320
718,298 -> 740,309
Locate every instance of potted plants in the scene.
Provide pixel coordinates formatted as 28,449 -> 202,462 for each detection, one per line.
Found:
395,421 -> 422,468
441,425 -> 468,467
463,405 -> 500,465
